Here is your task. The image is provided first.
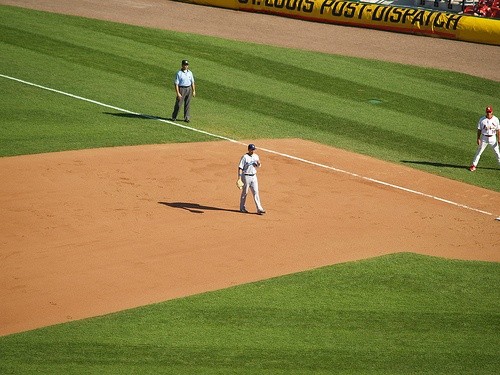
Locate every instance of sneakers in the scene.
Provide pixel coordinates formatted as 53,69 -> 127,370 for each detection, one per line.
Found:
240,208 -> 266,215
468,165 -> 476,172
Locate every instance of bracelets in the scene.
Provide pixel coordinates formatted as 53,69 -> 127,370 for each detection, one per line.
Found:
239,174 -> 242,176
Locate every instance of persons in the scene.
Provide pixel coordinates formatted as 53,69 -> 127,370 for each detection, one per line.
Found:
468,106 -> 500,172
238,144 -> 266,214
472,0 -> 500,20
171,60 -> 196,123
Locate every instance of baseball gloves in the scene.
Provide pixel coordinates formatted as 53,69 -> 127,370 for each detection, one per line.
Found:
236,180 -> 244,189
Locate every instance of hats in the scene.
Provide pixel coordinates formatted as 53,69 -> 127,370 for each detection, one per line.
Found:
248,144 -> 256,150
486,107 -> 493,113
182,60 -> 189,66
